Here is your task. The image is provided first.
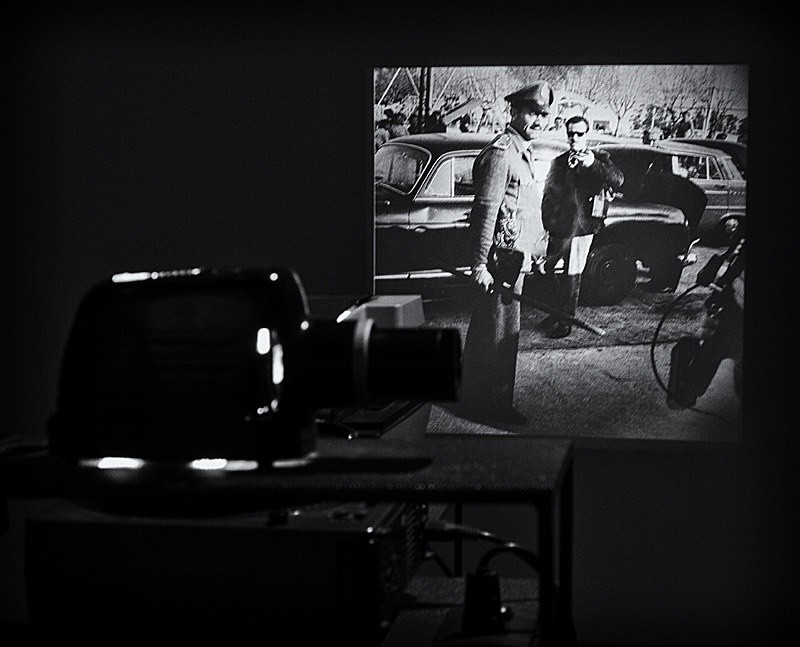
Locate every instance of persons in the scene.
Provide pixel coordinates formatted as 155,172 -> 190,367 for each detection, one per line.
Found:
540,115 -> 624,339
374,109 -> 447,151
646,106 -> 747,143
462,79 -> 554,425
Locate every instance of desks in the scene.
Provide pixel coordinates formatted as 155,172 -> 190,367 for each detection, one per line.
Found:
0,400 -> 578,647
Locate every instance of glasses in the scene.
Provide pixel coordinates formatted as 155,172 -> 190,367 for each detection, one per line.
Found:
568,130 -> 587,136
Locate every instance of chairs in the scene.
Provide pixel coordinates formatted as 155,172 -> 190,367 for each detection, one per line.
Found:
46,263 -> 318,451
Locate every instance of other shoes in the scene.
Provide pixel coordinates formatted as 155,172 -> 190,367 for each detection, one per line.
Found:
490,407 -> 528,425
552,325 -> 571,338
541,315 -> 558,327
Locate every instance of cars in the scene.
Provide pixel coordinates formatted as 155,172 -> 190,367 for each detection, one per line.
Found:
374,131 -> 495,287
533,130 -> 749,303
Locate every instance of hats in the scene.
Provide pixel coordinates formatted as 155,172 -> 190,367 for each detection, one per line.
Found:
504,81 -> 554,116
